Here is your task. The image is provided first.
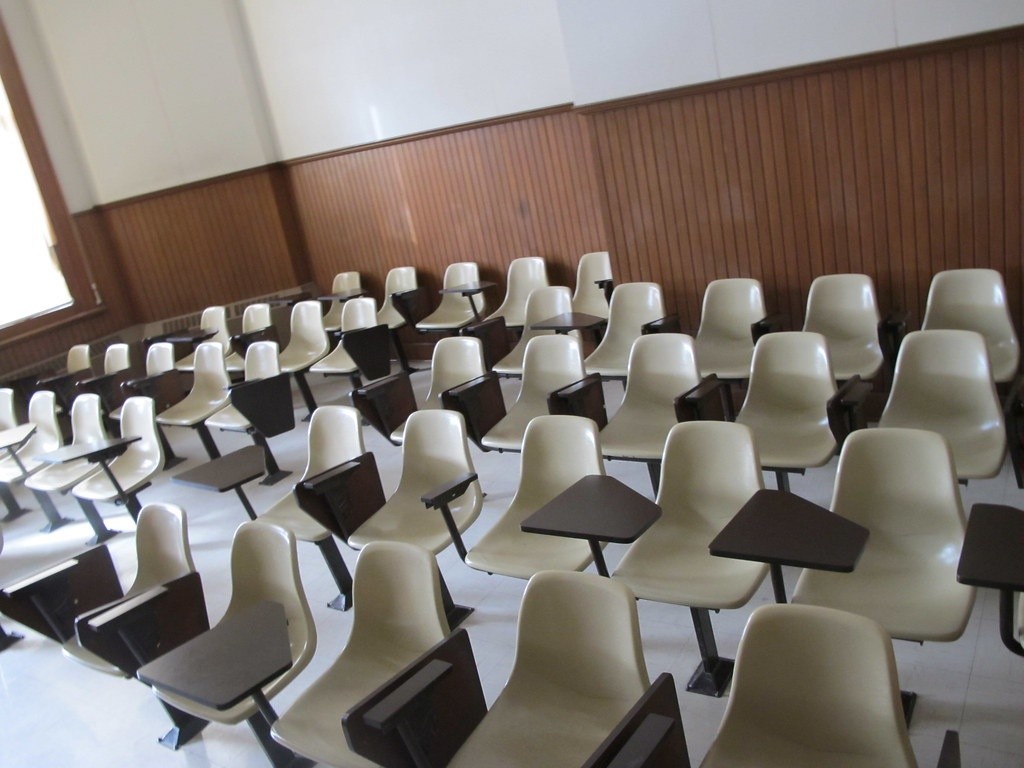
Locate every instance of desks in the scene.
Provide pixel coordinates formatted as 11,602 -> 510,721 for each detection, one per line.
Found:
709,489 -> 871,603
521,474 -> 662,578
317,288 -> 368,303
165,328 -> 219,343
438,281 -> 497,322
136,601 -> 292,724
957,502 -> 1024,657
0,422 -> 37,476
32,436 -> 142,505
270,292 -> 311,306
171,446 -> 265,519
530,312 -> 606,335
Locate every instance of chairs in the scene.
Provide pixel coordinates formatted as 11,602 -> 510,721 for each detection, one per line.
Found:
0,251 -> 1024,768
